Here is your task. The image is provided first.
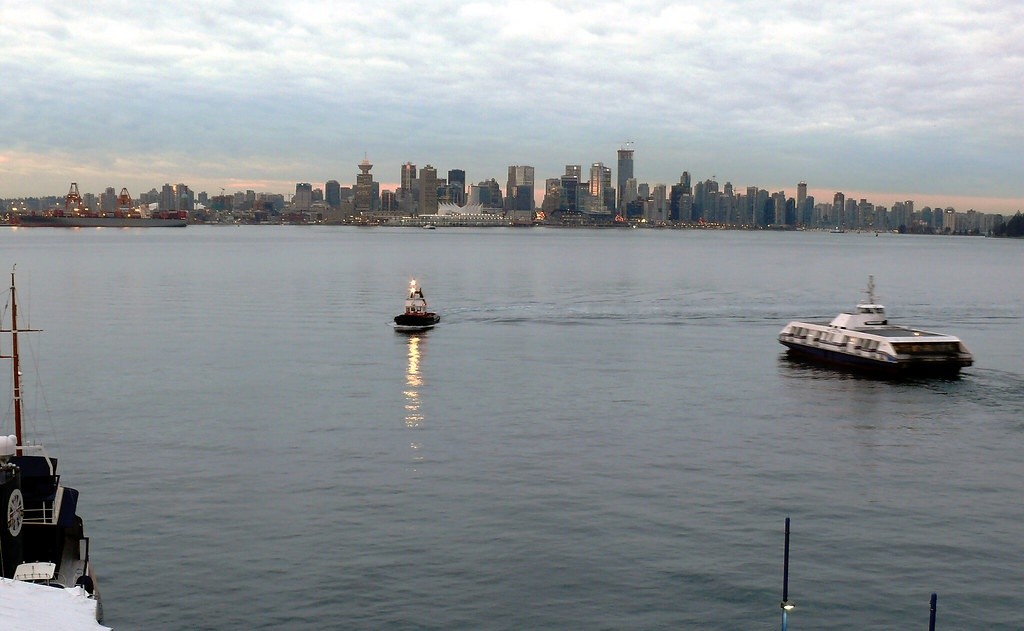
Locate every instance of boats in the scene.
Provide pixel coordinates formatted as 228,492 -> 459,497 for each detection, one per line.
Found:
388,286 -> 441,336
13,180 -> 190,227
1,263 -> 113,631
776,273 -> 974,372
